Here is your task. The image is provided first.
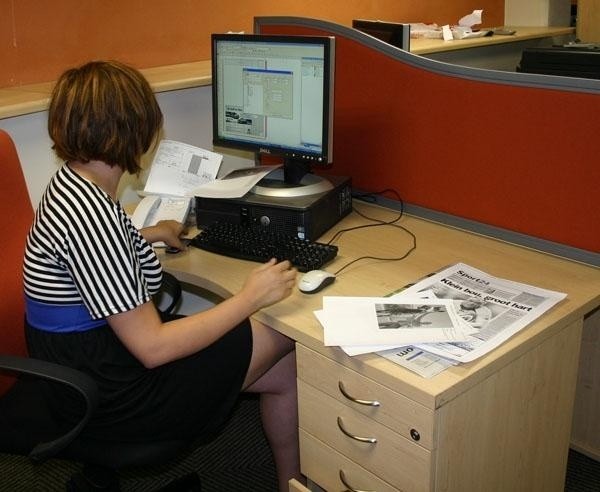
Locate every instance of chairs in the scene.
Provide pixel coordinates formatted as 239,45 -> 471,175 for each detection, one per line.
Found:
0,128 -> 240,491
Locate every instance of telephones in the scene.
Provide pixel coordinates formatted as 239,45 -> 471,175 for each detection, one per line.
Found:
131,195 -> 192,248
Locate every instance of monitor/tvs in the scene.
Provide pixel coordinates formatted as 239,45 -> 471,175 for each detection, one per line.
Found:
207,32 -> 337,197
351,20 -> 411,52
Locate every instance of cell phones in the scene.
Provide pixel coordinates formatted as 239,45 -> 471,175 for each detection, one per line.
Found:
164,238 -> 192,254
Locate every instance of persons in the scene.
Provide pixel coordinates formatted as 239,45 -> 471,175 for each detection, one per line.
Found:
457,297 -> 485,322
21,60 -> 306,492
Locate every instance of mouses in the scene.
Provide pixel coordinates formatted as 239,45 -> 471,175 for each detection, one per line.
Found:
298,268 -> 336,294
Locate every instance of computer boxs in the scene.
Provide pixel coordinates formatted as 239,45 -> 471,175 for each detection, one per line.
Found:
194,174 -> 351,241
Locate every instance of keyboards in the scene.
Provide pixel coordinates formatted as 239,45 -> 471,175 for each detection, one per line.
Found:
192,219 -> 339,273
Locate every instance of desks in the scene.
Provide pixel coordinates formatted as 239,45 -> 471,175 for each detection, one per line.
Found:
122,198 -> 600,492
